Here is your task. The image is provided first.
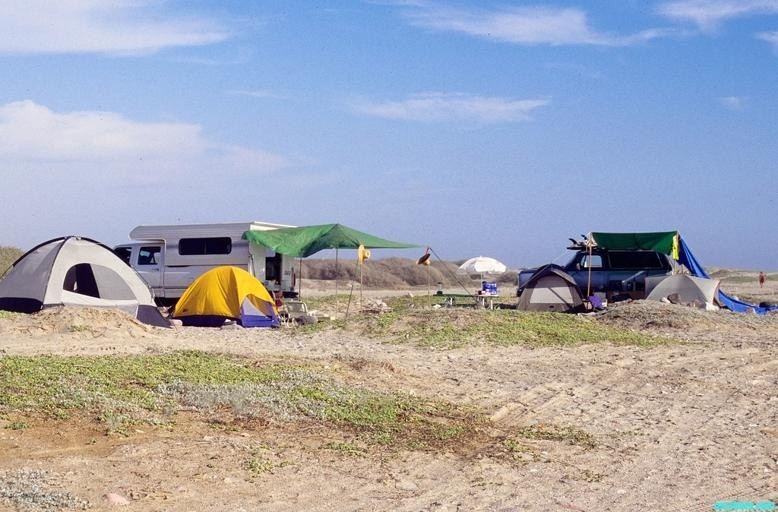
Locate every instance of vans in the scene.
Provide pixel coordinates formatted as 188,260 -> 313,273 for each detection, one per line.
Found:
110,220 -> 298,300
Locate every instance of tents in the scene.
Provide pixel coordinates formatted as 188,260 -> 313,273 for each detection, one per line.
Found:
165,266 -> 281,328
0,236 -> 172,328
517,264 -> 586,313
644,271 -> 723,311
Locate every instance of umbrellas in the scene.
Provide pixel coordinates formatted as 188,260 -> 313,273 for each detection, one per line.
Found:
456,256 -> 507,291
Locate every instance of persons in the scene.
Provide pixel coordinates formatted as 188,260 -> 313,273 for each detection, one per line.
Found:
759,271 -> 765,287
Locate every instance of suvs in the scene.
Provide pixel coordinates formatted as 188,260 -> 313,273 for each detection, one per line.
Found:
515,247 -> 679,303
